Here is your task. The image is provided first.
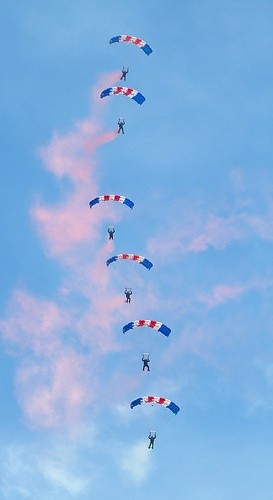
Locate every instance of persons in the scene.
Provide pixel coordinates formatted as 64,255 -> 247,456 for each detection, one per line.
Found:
120,66 -> 129,81
147,432 -> 156,449
108,227 -> 115,240
117,118 -> 125,134
142,355 -> 151,371
124,288 -> 133,304
